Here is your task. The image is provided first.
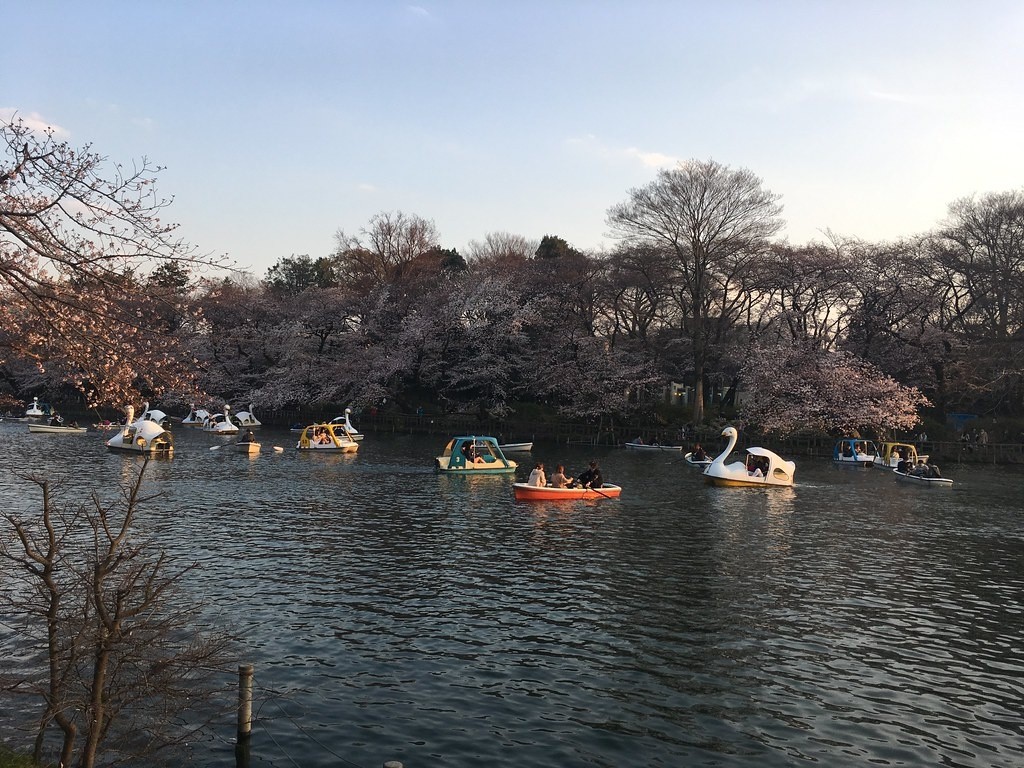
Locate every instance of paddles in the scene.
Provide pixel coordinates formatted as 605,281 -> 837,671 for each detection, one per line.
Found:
705,453 -> 714,460
899,472 -> 910,481
657,445 -> 667,453
665,456 -> 692,465
256,442 -> 283,451
573,479 -> 611,499
209,441 -> 240,451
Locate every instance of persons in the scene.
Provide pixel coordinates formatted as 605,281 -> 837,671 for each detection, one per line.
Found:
416,405 -> 425,424
576,460 -> 604,488
103,416 -> 126,425
50,414 -> 64,426
749,458 -> 769,476
843,442 -> 877,457
370,400 -> 378,428
898,455 -> 941,479
241,427 -> 256,443
890,446 -> 907,458
692,442 -> 707,461
960,427 -> 989,455
466,443 -> 487,463
635,435 -> 644,445
648,434 -> 659,445
913,431 -> 928,453
315,426 -> 344,444
551,464 -> 573,489
528,462 -> 546,487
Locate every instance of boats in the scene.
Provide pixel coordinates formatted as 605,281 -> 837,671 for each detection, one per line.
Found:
625,442 -> 682,451
234,405 -> 263,427
909,455 -> 929,465
296,423 -> 359,454
290,428 -> 313,433
872,442 -> 919,472
325,408 -> 365,441
0,397 -> 126,433
513,483 -> 622,501
702,427 -> 796,487
137,401 -> 172,430
892,465 -> 954,487
107,405 -> 173,453
472,443 -> 533,451
833,439 -> 880,467
203,404 -> 239,434
684,453 -> 713,468
181,410 -> 210,427
434,435 -> 518,474
234,442 -> 262,453
